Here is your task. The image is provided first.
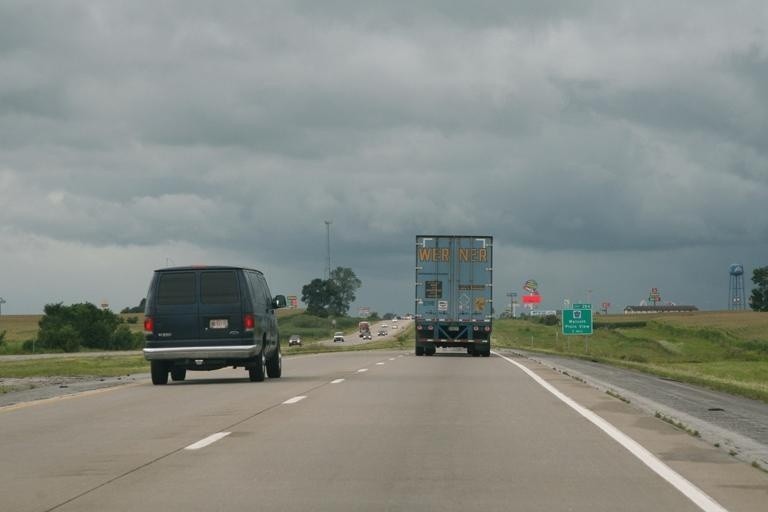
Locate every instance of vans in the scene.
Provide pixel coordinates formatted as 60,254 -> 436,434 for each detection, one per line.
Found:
143,265 -> 280,383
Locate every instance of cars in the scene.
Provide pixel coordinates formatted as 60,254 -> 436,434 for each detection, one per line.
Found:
289,335 -> 302,346
378,330 -> 387,336
334,331 -> 344,342
363,332 -> 371,339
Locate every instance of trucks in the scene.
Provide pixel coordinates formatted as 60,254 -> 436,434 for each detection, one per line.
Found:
415,235 -> 494,356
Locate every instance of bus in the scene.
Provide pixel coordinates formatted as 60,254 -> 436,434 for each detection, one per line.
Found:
359,322 -> 369,337
